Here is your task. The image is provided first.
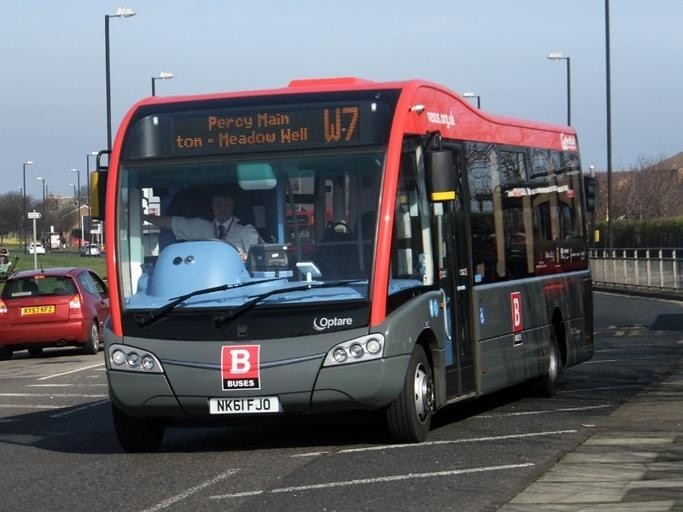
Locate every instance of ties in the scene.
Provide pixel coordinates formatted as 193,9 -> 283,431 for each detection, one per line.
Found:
218,225 -> 225,239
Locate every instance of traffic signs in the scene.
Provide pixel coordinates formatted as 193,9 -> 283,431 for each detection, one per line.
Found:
27,212 -> 42,219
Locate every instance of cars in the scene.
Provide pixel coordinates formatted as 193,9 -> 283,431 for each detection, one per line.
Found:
0,266 -> 110,360
0,246 -> 19,282
25,239 -> 46,255
70,238 -> 101,257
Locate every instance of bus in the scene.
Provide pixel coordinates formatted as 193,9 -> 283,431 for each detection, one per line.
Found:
43,232 -> 61,249
89,77 -> 594,451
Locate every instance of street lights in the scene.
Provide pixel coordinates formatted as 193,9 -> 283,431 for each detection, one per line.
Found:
18,161 -> 33,255
87,152 -> 99,258
68,168 -> 81,251
105,7 -> 136,169
152,72 -> 175,96
463,92 -> 480,109
547,52 -> 570,127
33,177 -> 48,250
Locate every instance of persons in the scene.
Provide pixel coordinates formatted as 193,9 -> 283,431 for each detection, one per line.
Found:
143,189 -> 258,259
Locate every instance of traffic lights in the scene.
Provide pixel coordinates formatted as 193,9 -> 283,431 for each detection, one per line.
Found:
584,175 -> 599,213
